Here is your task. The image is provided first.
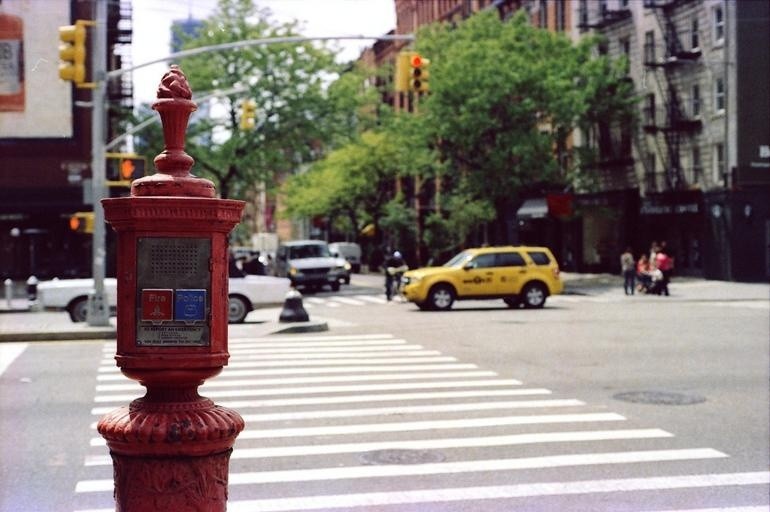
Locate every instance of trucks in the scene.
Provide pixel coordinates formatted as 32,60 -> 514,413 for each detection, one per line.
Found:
254,234 -> 279,255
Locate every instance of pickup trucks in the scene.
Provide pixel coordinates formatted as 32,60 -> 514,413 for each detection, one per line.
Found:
36,259 -> 291,323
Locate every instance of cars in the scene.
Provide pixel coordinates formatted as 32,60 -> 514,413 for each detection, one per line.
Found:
232,241 -> 362,291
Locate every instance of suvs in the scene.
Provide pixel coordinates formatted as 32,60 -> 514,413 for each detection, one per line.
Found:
400,245 -> 563,308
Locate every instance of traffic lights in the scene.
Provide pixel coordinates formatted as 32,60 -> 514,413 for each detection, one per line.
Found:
241,101 -> 257,127
70,212 -> 84,233
393,48 -> 432,95
105,154 -> 147,188
59,19 -> 95,86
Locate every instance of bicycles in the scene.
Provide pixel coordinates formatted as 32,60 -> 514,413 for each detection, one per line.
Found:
385,274 -> 401,300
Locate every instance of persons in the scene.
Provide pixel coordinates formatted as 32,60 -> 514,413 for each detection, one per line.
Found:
241,251 -> 287,277
383,251 -> 409,301
619,240 -> 674,296
